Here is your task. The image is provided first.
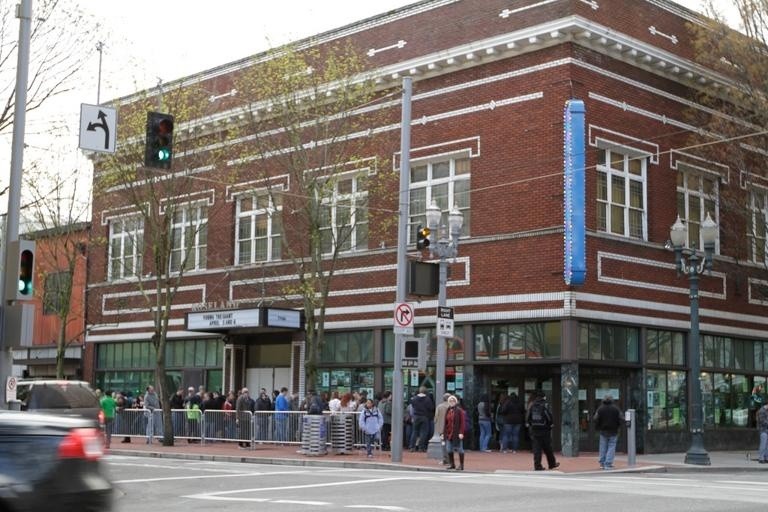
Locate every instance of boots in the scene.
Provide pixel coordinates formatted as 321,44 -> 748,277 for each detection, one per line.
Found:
446,451 -> 455,469
456,453 -> 464,469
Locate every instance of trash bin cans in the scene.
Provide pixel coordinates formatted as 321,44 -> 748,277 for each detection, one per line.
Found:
330,415 -> 354,455
296,414 -> 328,457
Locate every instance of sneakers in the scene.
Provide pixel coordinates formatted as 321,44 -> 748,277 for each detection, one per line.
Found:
535,467 -> 545,470
600,463 -> 615,469
121,440 -> 130,443
238,444 -> 250,450
549,463 -> 560,470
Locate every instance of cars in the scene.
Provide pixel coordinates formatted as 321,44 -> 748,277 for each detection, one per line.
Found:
1,377 -> 117,510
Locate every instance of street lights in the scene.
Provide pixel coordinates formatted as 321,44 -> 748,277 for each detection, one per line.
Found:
668,206 -> 719,466
426,196 -> 461,460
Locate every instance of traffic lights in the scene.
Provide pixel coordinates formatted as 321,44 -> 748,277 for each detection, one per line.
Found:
415,226 -> 431,249
16,240 -> 36,301
145,111 -> 174,174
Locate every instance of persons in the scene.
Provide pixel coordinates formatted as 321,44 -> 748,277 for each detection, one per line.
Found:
402,385 -> 526,469
526,391 -> 560,471
592,394 -> 624,470
94,385 -> 392,456
755,403 -> 768,463
